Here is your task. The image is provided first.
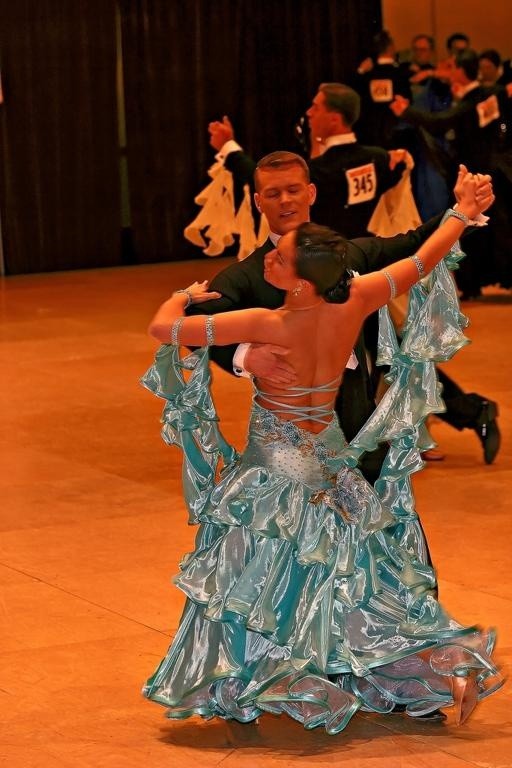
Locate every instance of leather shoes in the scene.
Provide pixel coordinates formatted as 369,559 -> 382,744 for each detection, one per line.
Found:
421,449 -> 445,461
473,397 -> 500,465
390,700 -> 447,722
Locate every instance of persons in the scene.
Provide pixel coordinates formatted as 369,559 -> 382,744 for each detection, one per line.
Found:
182,150 -> 497,724
141,163 -> 508,745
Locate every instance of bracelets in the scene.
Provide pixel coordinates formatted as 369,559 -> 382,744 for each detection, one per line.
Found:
178,287 -> 193,310
